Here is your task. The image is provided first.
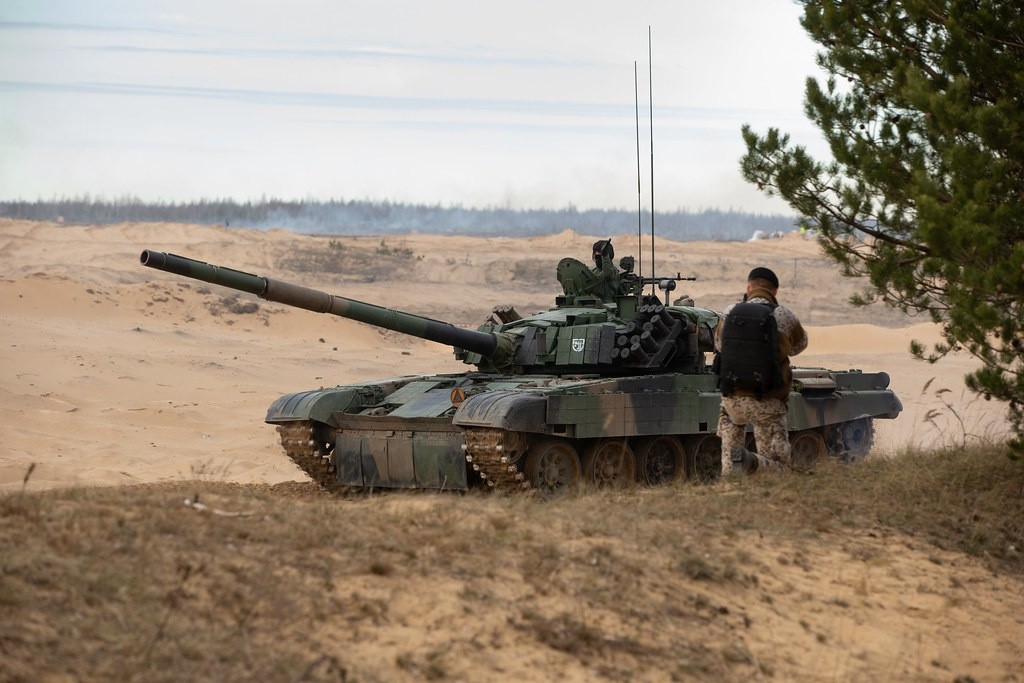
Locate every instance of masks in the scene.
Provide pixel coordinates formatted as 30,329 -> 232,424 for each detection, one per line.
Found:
595,254 -> 602,267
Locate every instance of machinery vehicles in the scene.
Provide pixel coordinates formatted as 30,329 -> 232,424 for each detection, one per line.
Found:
138,238 -> 906,496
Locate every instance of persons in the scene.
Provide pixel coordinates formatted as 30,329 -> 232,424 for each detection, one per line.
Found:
714,268 -> 810,481
590,238 -> 620,303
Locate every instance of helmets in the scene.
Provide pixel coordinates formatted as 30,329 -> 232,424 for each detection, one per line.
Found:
592,240 -> 614,259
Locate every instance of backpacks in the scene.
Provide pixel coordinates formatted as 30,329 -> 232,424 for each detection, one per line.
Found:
711,301 -> 789,403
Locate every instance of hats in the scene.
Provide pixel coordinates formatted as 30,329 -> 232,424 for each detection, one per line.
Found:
748,267 -> 779,289
